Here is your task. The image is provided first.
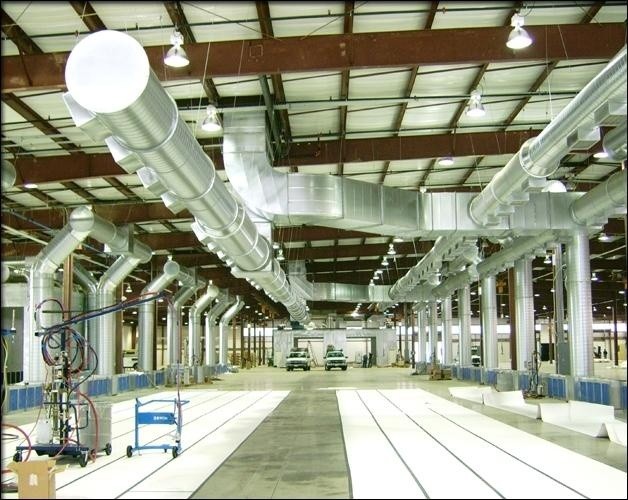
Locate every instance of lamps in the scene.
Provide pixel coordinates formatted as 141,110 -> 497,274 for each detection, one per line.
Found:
467,86 -> 485,115
368,269 -> 383,287
505,13 -> 532,50
203,99 -> 224,133
276,248 -> 286,261
387,244 -> 396,256
163,17 -> 190,68
382,256 -> 390,267
126,284 -> 132,292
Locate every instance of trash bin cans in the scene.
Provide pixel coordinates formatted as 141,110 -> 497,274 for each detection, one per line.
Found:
79,402 -> 111,455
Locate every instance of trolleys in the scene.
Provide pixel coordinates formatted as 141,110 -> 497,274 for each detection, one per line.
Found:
126,398 -> 190,458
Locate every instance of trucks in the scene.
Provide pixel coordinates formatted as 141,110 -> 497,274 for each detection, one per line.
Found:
457,346 -> 481,367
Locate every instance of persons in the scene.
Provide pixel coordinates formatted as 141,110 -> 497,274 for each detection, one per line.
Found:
363,353 -> 368,368
603,349 -> 607,359
368,352 -> 373,368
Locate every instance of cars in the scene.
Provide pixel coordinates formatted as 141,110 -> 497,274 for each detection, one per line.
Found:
285,351 -> 311,371
323,351 -> 348,370
123,349 -> 138,370
